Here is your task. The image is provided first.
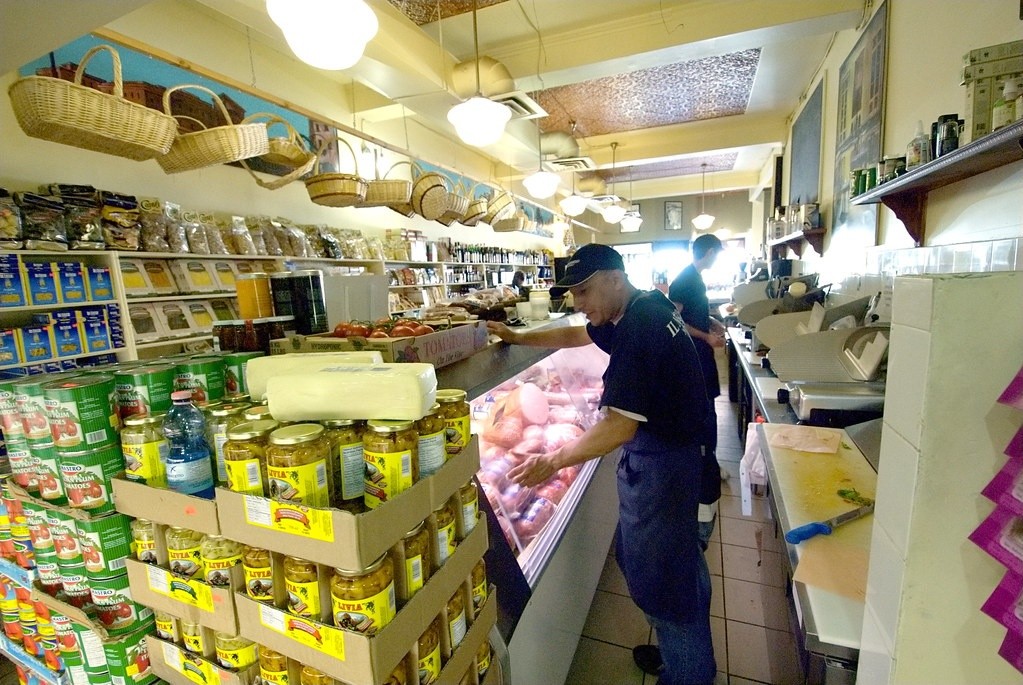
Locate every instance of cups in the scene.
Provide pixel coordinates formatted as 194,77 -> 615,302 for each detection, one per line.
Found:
530,297 -> 548,319
516,302 -> 531,318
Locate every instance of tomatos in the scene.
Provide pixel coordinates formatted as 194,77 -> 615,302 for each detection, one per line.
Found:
334,318 -> 434,338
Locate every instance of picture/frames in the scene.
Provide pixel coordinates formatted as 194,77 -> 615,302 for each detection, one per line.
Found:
664,201 -> 683,231
619,203 -> 641,234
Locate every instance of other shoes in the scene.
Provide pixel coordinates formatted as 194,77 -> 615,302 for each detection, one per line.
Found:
632,644 -> 667,676
720,467 -> 731,480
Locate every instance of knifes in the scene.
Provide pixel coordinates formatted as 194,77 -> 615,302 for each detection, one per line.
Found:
785,501 -> 874,544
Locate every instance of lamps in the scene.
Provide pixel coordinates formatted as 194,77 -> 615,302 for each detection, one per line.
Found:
691,163 -> 716,229
714,191 -> 732,241
522,90 -> 562,200
559,172 -> 589,217
620,166 -> 643,232
447,0 -> 513,148
265,0 -> 379,70
601,142 -> 627,224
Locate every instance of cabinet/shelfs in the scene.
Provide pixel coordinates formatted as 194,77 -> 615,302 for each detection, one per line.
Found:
0,248 -> 561,382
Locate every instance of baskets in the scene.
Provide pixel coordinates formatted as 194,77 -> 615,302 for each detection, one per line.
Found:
258,119 -> 309,168
7,44 -> 179,161
234,112 -> 317,190
304,137 -> 372,209
154,84 -> 269,176
354,149 -> 413,206
382,161 -> 538,234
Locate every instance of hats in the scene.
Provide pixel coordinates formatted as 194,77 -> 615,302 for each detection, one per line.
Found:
692,233 -> 725,252
549,243 -> 624,297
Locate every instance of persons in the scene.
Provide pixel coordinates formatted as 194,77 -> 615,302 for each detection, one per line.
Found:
668,234 -> 731,481
733,262 -> 747,283
511,270 -> 530,302
486,244 -> 717,685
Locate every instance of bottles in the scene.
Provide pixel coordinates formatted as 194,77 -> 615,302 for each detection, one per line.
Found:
385,227 -> 555,298
162,391 -> 214,499
849,113 -> 965,200
270,272 -> 296,331
234,273 -> 273,318
291,270 -> 329,335
767,194 -> 820,241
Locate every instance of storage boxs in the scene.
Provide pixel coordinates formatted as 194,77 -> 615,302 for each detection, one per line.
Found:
245,350 -> 384,402
774,205 -> 786,221
265,362 -> 438,422
269,319 -> 488,371
108,432 -> 504,685
800,203 -> 819,230
959,38 -> 1023,146
386,228 -> 438,262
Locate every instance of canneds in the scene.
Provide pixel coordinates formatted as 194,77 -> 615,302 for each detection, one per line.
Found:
193,389 -> 470,509
0,457 -> 170,685
131,476 -> 492,685
212,268 -> 331,357
0,350 -> 264,516
